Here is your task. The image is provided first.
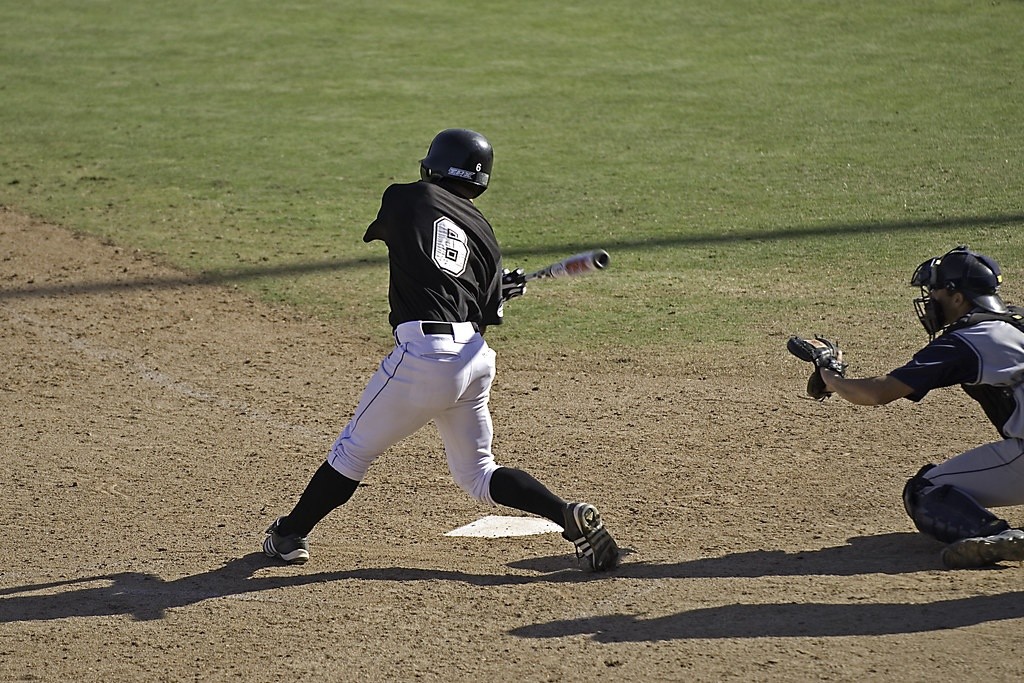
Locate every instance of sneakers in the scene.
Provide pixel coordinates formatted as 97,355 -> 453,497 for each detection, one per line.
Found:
941,528 -> 1024,570
561,502 -> 620,571
262,515 -> 310,563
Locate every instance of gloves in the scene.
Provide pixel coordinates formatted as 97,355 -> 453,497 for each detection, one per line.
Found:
502,265 -> 527,302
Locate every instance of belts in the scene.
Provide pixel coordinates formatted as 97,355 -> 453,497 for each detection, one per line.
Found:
394,322 -> 486,345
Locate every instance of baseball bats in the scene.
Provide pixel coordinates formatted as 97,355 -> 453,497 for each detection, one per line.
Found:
521,247 -> 612,284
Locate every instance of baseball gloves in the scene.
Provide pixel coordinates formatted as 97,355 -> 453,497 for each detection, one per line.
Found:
786,334 -> 846,401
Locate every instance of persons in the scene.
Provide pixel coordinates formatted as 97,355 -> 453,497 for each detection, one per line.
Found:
261,129 -> 621,569
787,245 -> 1024,567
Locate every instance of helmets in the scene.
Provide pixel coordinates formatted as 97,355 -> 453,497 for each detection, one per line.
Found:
417,128 -> 494,199
910,245 -> 1009,314
